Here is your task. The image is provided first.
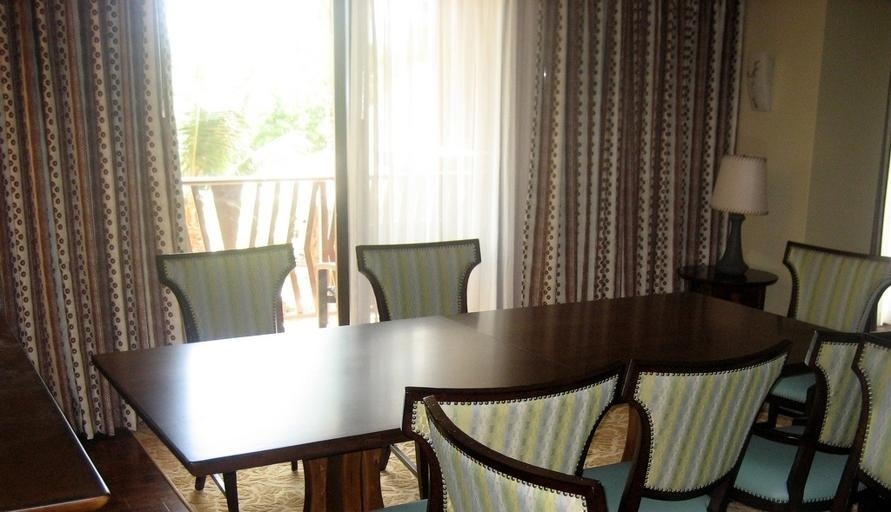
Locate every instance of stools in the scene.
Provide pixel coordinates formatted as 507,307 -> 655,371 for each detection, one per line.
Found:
317,261 -> 339,328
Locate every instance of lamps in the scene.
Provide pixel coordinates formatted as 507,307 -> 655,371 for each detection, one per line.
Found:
709,154 -> 770,274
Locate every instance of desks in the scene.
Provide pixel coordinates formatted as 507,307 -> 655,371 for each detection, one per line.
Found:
90,291 -> 850,512
678,265 -> 778,311
0,314 -> 113,512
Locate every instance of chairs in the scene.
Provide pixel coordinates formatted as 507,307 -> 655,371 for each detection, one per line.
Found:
422,394 -> 604,512
373,371 -> 621,512
765,241 -> 891,429
584,336 -> 795,512
356,237 -> 482,322
851,333 -> 891,512
730,331 -> 865,512
157,245 -> 298,490
